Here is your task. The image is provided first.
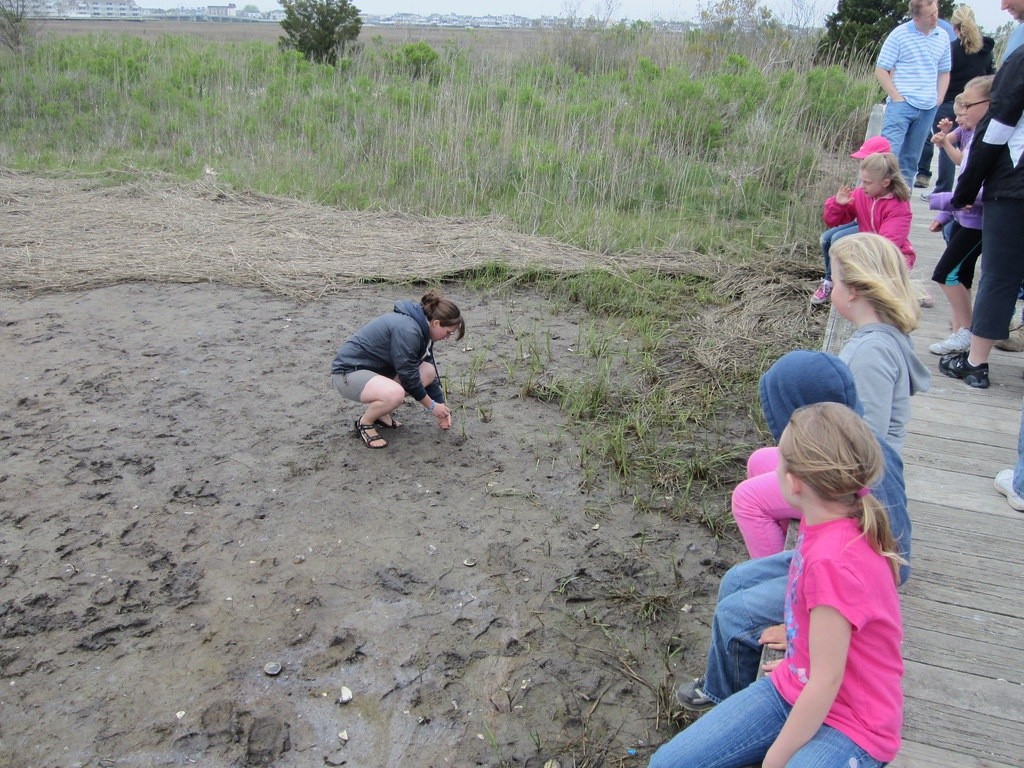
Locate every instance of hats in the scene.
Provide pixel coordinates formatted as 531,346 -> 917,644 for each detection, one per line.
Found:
850,136 -> 890,159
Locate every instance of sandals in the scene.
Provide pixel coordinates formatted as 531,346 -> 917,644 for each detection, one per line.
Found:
364,411 -> 403,430
355,415 -> 388,448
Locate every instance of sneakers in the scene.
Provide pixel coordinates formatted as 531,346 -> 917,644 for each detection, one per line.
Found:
994,469 -> 1024,511
810,277 -> 833,304
1008,299 -> 1024,331
938,349 -> 989,388
929,326 -> 971,355
676,672 -> 717,711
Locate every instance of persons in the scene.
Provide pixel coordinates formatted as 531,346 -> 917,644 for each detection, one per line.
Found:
993,395 -> 1024,511
673,351 -> 913,714
330,292 -> 466,449
880,0 -> 951,198
809,135 -> 891,304
821,150 -> 917,271
827,230 -> 932,459
914,0 -> 1024,388
646,402 -> 905,768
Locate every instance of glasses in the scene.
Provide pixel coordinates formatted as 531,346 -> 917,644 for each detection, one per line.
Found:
961,99 -> 991,110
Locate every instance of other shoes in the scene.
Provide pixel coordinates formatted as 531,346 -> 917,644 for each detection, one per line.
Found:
920,191 -> 932,202
993,325 -> 1024,352
914,174 -> 930,188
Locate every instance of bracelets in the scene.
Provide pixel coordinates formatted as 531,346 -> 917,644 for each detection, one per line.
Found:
429,400 -> 436,412
